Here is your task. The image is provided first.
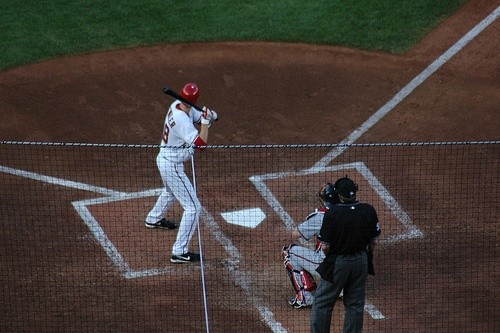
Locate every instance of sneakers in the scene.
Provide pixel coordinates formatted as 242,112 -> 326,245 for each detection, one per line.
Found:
171,252 -> 200,262
146,217 -> 177,229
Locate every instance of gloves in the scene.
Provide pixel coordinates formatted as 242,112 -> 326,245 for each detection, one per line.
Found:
201,106 -> 212,125
211,111 -> 217,121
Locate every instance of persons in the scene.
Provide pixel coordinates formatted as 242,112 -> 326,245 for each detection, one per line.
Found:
145,83 -> 216,263
309,177 -> 382,333
282,183 -> 344,308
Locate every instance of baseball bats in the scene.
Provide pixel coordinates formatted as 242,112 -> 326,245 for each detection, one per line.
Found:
162,88 -> 217,121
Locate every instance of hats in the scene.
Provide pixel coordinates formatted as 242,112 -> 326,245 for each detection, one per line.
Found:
334,177 -> 356,197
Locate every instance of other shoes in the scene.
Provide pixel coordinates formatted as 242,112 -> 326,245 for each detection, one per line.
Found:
288,298 -> 308,308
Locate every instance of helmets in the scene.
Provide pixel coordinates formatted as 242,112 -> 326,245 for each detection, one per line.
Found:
319,183 -> 337,203
179,82 -> 199,104
